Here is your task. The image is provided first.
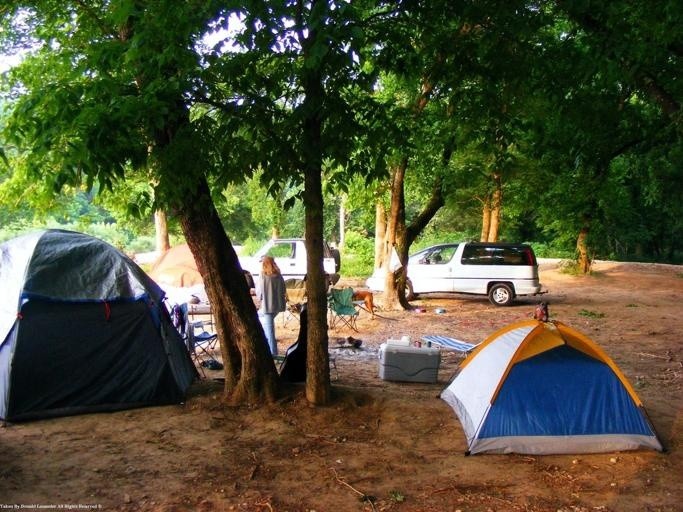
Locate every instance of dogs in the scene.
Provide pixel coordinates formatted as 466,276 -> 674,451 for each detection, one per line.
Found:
353,291 -> 376,320
534,302 -> 551,323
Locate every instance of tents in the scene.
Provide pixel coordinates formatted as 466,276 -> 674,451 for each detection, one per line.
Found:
435,301 -> 664,455
0,228 -> 201,422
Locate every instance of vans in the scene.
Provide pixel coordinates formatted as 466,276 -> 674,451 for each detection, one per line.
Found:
365,241 -> 541,308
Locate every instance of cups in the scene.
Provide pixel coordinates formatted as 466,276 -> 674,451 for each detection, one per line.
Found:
416,342 -> 421,347
426,341 -> 431,347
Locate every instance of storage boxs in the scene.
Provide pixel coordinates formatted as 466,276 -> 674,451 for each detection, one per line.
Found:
380,342 -> 439,384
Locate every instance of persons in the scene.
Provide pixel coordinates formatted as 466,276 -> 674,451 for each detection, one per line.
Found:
242,256 -> 286,356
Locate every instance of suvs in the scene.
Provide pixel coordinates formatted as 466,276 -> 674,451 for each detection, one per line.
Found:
238,239 -> 341,291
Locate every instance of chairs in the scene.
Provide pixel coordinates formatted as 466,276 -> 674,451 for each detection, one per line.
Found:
171,286 -> 360,379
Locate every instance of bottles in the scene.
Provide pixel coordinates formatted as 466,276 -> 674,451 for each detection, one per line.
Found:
173,304 -> 180,327
